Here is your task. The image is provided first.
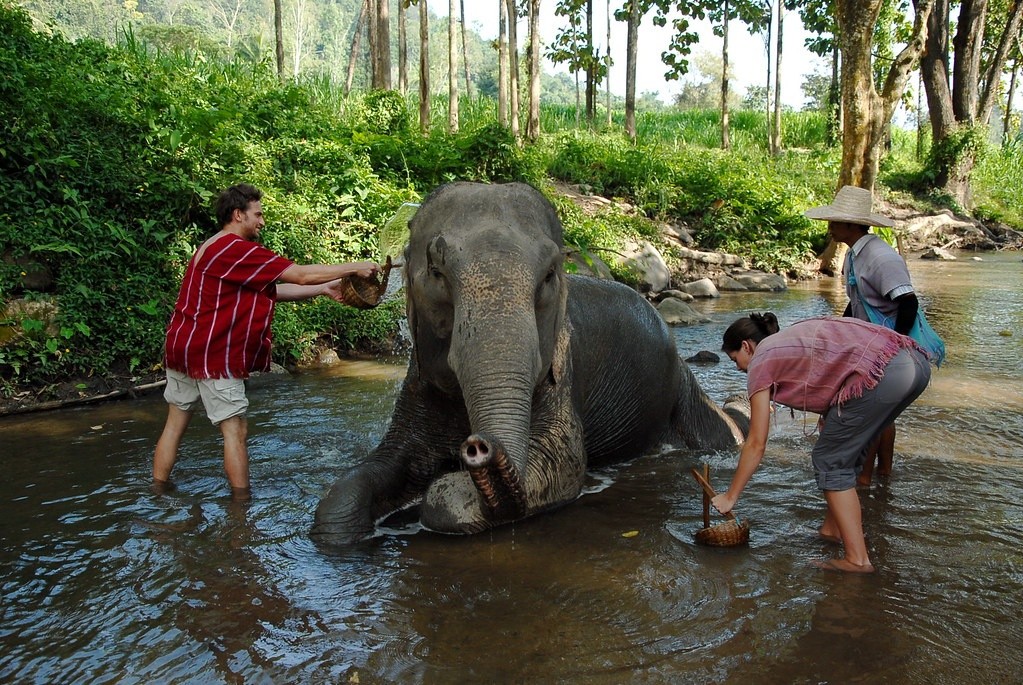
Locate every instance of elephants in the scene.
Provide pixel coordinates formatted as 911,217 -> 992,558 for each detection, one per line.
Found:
307,180 -> 777,550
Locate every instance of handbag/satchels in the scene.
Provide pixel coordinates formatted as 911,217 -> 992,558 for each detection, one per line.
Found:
862,299 -> 945,371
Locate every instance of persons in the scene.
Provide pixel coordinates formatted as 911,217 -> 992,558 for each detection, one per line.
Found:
804,186 -> 919,486
154,183 -> 383,488
709,311 -> 930,565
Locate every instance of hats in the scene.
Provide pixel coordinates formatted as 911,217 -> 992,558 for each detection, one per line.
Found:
805,185 -> 895,228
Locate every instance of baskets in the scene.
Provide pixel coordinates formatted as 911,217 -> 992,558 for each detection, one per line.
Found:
340,255 -> 404,309
690,461 -> 750,547
